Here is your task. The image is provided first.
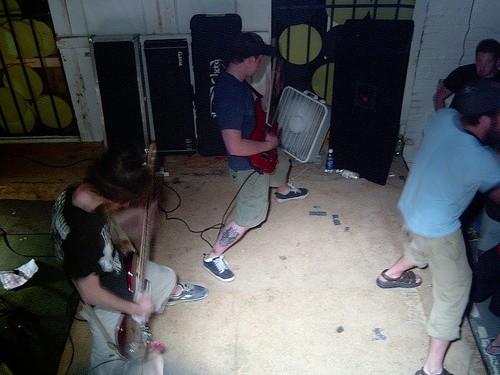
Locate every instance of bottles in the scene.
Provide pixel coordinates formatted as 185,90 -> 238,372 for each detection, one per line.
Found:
395,133 -> 404,156
325,148 -> 334,173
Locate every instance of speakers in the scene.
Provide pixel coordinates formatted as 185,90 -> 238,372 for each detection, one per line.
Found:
328,18 -> 414,185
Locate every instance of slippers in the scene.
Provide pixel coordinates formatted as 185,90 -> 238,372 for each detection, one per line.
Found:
486,338 -> 500,355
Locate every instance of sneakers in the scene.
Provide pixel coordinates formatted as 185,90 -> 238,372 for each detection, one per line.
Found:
202,253 -> 237,281
165,282 -> 210,305
274,183 -> 309,203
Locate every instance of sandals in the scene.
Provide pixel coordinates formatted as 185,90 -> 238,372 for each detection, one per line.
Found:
415,366 -> 455,375
376,268 -> 423,289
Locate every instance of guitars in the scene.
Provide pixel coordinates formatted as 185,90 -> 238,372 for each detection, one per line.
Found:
246,57 -> 282,174
113,142 -> 160,362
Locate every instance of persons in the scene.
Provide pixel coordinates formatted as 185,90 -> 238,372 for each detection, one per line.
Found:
50,149 -> 208,375
376,83 -> 500,375
202,31 -> 310,281
434,38 -> 500,117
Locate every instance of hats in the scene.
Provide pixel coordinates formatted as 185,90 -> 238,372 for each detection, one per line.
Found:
225,31 -> 280,60
